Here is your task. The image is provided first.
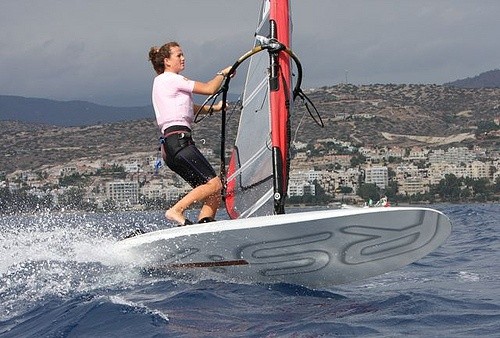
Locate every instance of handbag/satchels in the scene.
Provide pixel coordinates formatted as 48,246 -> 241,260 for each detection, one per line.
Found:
158,135 -> 167,162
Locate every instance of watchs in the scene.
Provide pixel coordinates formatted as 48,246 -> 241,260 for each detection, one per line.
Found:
216,71 -> 225,77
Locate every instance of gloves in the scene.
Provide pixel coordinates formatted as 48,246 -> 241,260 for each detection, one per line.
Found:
216,65 -> 236,78
208,99 -> 229,112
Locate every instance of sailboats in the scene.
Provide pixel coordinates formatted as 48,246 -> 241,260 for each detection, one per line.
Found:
118,1 -> 451,286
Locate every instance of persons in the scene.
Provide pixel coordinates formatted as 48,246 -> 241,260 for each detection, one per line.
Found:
368,194 -> 391,207
148,41 -> 237,226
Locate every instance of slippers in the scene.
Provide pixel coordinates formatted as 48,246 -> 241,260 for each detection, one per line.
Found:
177,217 -> 217,227
